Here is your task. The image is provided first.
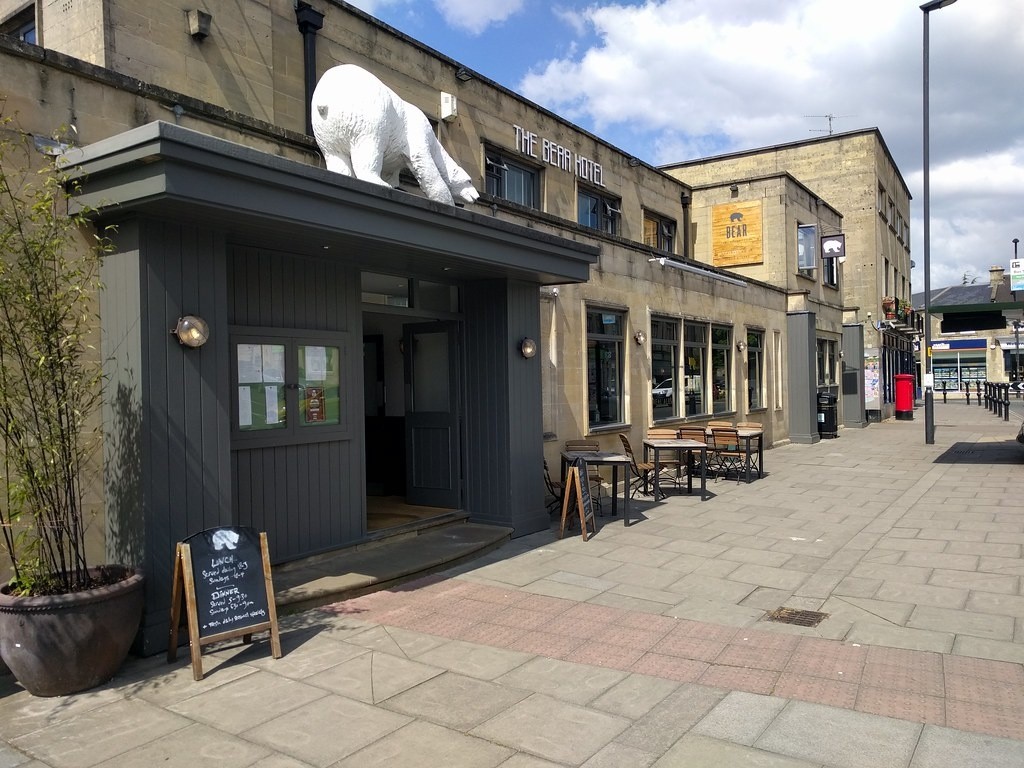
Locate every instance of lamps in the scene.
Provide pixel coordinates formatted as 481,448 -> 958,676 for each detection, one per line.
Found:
456,68 -> 473,82
520,337 -> 537,359
634,330 -> 647,345
169,315 -> 210,347
628,158 -> 640,167
737,341 -> 746,352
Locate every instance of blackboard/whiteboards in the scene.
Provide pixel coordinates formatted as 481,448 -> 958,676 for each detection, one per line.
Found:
174,524 -> 278,645
560,457 -> 595,523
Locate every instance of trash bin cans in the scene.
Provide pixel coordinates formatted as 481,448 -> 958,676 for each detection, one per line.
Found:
816,392 -> 839,438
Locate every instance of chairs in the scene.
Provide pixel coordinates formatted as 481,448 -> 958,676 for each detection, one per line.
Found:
544,420 -> 765,517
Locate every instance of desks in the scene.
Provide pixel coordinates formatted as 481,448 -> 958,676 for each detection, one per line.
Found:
677,430 -> 763,483
642,438 -> 708,502
561,451 -> 632,527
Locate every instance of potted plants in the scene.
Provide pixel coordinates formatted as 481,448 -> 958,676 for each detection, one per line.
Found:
898,300 -> 910,314
0,96 -> 146,698
886,307 -> 896,319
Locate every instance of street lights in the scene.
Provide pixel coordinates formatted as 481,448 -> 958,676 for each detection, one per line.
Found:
919,0 -> 962,444
